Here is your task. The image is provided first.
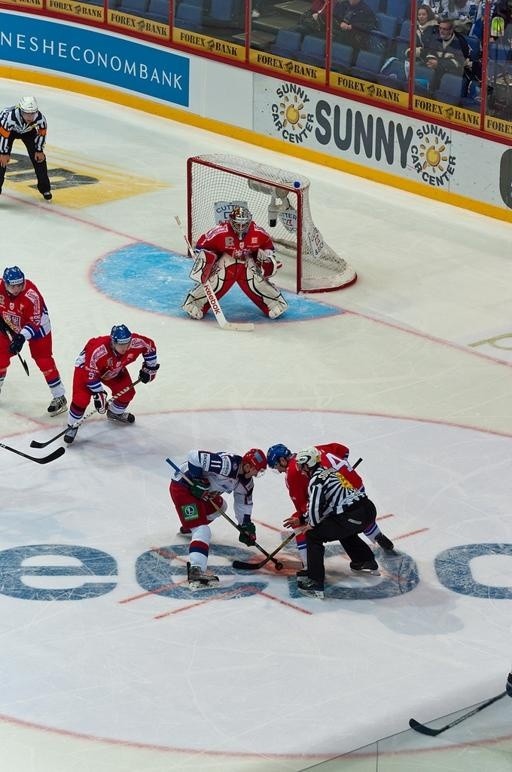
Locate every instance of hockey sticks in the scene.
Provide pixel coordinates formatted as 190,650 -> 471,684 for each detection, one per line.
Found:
233,459 -> 363,571
176,215 -> 255,332
166,457 -> 283,572
2,326 -> 30,377
0,445 -> 65,465
410,691 -> 507,736
30,377 -> 142,448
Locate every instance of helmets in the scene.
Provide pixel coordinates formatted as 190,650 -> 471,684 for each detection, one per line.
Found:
111,324 -> 132,352
229,206 -> 252,236
3,266 -> 26,296
16,96 -> 38,113
266,444 -> 290,469
242,449 -> 267,478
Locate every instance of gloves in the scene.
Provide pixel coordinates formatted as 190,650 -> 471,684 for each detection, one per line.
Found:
8,334 -> 25,355
239,522 -> 256,546
191,478 -> 211,502
92,390 -> 108,414
139,362 -> 160,384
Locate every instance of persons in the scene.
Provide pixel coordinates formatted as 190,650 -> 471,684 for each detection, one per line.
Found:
292,0 -> 337,33
170,448 -> 266,580
266,442 -> 394,570
0,266 -> 66,412
64,324 -> 158,444
401,0 -> 499,85
183,204 -> 288,321
297,447 -> 379,593
333,0 -> 376,46
0,95 -> 52,200
506,673 -> 512,697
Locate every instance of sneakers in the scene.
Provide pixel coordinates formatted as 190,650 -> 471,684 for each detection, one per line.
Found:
48,395 -> 67,412
180,527 -> 192,534
296,565 -> 324,591
64,424 -> 78,443
189,566 -> 219,584
350,557 -> 378,570
42,191 -> 52,200
107,410 -> 135,423
377,535 -> 393,551
